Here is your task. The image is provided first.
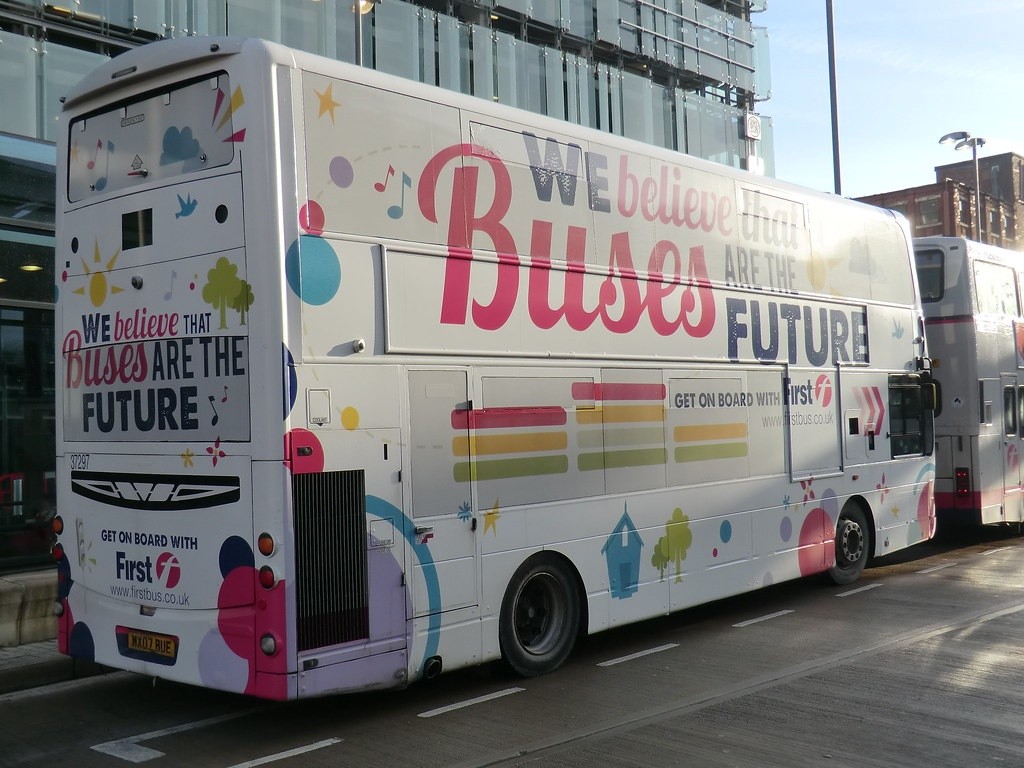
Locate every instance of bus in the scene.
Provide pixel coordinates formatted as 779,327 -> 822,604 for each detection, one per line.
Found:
911,235 -> 1024,532
43,35 -> 940,707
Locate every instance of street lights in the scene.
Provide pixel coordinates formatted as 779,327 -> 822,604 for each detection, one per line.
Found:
939,131 -> 985,243
